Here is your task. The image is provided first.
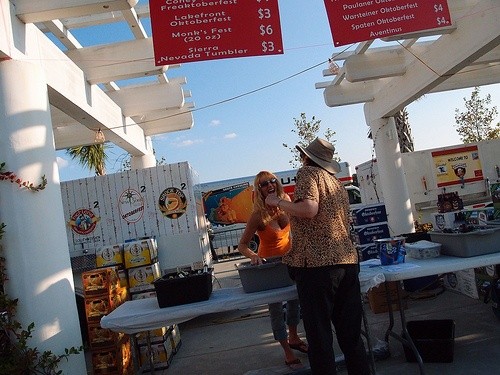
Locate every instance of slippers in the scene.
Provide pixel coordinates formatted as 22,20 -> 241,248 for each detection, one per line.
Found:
285,341 -> 309,370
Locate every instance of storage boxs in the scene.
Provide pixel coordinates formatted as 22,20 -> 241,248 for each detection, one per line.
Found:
151,268 -> 214,308
82,239 -> 182,375
237,256 -> 295,293
404,240 -> 442,259
427,183 -> 500,300
367,280 -> 408,314
401,319 -> 455,364
348,204 -> 391,262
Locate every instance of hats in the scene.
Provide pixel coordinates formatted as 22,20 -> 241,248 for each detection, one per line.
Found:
296,138 -> 341,175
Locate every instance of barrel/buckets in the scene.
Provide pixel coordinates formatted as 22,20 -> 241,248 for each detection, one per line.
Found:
375,238 -> 405,265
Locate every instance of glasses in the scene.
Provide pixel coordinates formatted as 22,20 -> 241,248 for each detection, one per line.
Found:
258,178 -> 277,187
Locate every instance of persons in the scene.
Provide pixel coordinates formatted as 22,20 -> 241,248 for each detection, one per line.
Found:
237,171 -> 309,370
205,214 -> 219,263
263,136 -> 374,375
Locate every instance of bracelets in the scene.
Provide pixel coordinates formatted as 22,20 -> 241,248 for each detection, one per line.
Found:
276,200 -> 282,207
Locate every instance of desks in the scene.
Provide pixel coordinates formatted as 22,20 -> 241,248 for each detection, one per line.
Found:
101,251 -> 500,375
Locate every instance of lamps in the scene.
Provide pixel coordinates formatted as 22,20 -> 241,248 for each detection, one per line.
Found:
328,58 -> 339,74
95,129 -> 105,143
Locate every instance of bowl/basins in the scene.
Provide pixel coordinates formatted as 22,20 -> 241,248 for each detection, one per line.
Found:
405,242 -> 442,259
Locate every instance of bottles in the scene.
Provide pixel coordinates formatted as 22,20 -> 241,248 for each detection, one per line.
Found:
437,188 -> 464,212
169,259 -> 209,279
